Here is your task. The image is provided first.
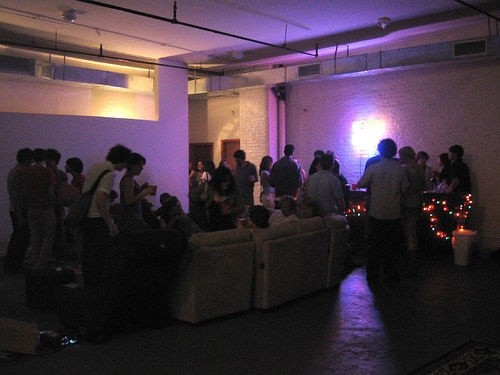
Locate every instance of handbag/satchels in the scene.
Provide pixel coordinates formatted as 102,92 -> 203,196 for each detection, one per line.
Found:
65,169 -> 111,225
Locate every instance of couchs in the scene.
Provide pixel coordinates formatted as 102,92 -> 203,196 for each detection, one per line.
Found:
171,214 -> 351,324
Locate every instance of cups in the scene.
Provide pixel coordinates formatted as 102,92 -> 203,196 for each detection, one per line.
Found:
148,184 -> 157,196
352,184 -> 356,190
344,184 -> 350,191
199,173 -> 202,179
237,218 -> 247,228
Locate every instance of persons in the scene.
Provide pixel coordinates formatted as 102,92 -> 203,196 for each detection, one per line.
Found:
109,145 -> 346,237
416,151 -> 433,192
436,153 -> 449,184
83,145 -> 131,296
357,139 -> 408,282
399,146 -> 421,276
2,148 -> 33,273
64,158 -> 86,223
45,150 -> 68,262
23,149 -> 58,269
445,145 -> 472,199
